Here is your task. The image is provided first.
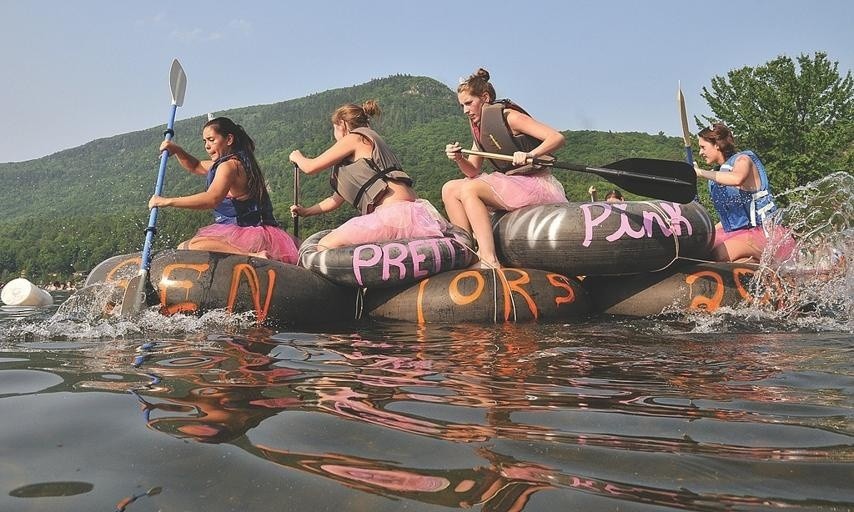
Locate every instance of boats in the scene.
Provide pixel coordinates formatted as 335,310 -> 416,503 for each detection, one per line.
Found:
294,226 -> 472,283
491,203 -> 715,268
694,258 -> 832,292
81,197 -> 854,336
368,271 -> 592,323
594,269 -> 761,317
56,251 -> 341,325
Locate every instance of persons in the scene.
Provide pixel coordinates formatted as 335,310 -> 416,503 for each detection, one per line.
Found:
289,99 -> 453,251
588,186 -> 625,202
693,123 -> 798,270
149,116 -> 300,267
441,66 -> 569,270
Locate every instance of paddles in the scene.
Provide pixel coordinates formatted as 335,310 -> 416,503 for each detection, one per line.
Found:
676,80 -> 701,204
447,143 -> 697,205
119,58 -> 188,315
